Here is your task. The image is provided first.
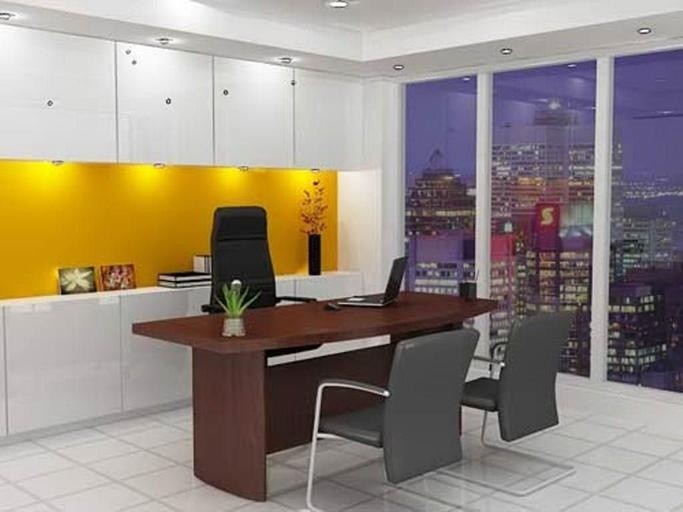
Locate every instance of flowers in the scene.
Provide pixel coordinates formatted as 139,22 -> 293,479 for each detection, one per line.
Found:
301,183 -> 328,234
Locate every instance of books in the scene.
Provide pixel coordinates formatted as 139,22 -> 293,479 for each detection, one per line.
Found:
156,271 -> 211,281
158,279 -> 211,288
209,256 -> 212,273
192,255 -> 205,273
205,255 -> 208,273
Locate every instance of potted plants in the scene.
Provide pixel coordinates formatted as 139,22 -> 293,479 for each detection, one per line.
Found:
214,281 -> 263,336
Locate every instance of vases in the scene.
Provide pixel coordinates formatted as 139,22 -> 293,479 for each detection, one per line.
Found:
309,235 -> 322,275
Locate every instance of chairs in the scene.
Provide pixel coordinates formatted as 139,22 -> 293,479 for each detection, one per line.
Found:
203,204 -> 318,314
436,310 -> 575,496
306,328 -> 482,511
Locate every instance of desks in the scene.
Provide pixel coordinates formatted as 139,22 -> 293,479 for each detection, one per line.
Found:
131,291 -> 501,503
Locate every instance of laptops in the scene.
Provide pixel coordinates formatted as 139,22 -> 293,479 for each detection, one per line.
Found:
337,256 -> 408,308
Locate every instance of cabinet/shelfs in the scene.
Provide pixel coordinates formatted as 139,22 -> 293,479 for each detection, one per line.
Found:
0,24 -> 113,165
276,273 -> 363,305
0,298 -> 121,434
295,71 -> 371,170
119,290 -> 210,413
115,43 -> 215,169
214,56 -> 293,172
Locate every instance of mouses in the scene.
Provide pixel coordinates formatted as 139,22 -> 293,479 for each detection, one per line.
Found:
324,302 -> 340,311
